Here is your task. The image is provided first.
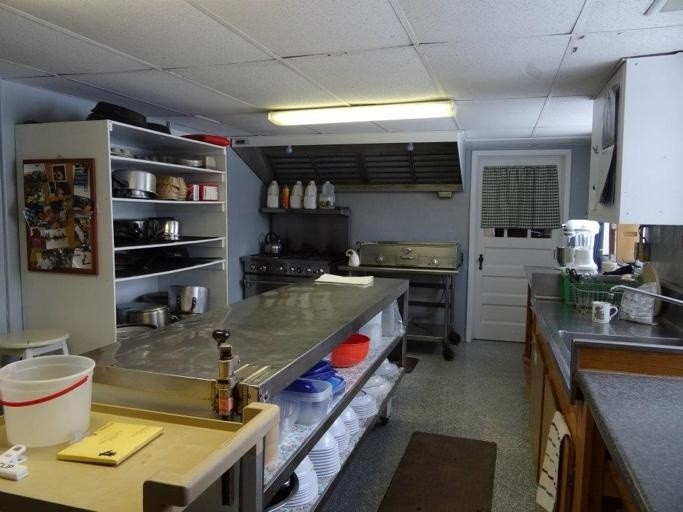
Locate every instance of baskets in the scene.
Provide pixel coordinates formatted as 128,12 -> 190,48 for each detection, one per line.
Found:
561,270 -> 641,308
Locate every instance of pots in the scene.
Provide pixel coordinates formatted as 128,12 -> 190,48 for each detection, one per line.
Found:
86,102 -> 223,343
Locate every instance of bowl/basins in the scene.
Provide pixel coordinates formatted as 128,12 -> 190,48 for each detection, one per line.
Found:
262,471 -> 299,511
323,332 -> 371,368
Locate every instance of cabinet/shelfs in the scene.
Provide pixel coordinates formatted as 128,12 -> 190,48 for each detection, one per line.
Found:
520,283 -> 643,511
16,119 -> 228,355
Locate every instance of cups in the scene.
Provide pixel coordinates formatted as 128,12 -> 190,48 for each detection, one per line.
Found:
603,262 -> 617,272
591,300 -> 619,323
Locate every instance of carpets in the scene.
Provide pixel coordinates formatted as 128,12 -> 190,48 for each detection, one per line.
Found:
371,431 -> 496,509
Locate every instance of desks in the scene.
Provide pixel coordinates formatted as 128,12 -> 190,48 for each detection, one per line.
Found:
1,401 -> 279,512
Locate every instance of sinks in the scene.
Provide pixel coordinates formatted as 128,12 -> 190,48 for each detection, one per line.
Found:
558,330 -> 683,353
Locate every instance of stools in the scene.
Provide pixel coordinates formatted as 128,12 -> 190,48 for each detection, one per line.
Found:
0,330 -> 71,365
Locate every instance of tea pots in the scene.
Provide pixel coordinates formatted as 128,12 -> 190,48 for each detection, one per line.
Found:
264,231 -> 283,253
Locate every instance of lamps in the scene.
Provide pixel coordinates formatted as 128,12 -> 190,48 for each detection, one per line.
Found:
268,99 -> 457,127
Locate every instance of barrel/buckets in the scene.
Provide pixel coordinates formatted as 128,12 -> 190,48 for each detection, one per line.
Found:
268,179 -> 336,210
0,355 -> 96,448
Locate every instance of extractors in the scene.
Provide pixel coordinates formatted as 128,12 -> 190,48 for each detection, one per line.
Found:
226,130 -> 467,199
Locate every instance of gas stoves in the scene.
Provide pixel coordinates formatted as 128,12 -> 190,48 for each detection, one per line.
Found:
241,253 -> 349,278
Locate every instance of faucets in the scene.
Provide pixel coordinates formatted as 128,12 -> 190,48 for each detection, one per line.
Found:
607,286 -> 683,306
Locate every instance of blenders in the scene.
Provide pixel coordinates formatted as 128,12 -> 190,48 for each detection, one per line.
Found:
560,219 -> 600,276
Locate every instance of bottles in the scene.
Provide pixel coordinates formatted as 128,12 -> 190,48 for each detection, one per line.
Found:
281,183 -> 289,208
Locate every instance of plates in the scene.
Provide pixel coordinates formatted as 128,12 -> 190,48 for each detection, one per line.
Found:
282,358 -> 398,507
